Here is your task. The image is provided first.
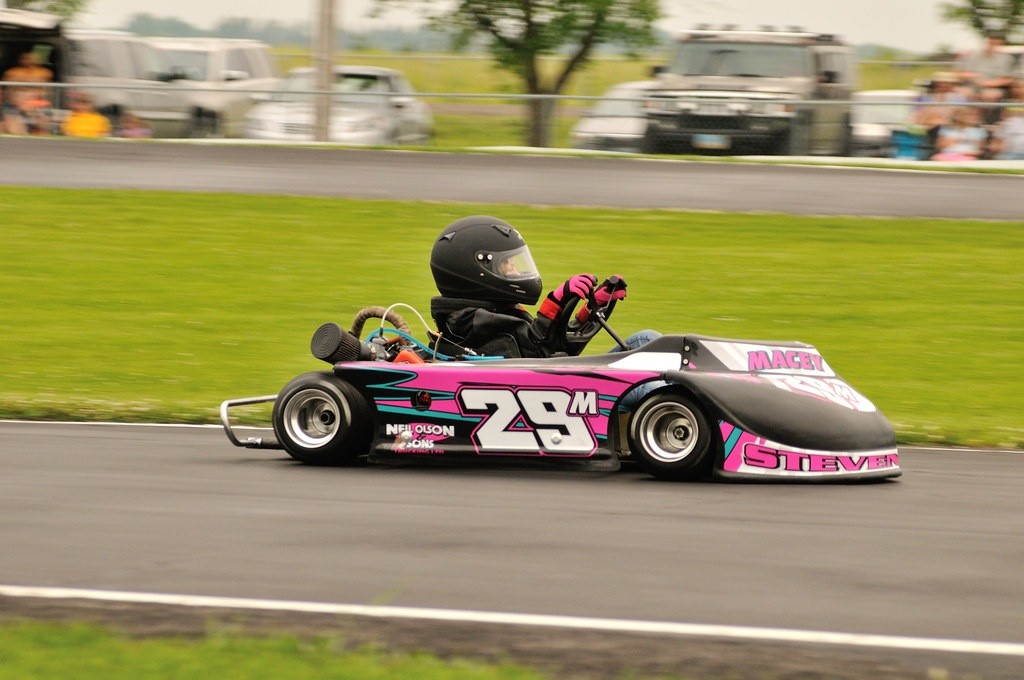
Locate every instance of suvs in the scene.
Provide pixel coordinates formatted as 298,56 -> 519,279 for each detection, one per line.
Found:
143,34 -> 274,137
0,8 -> 205,139
640,23 -> 855,156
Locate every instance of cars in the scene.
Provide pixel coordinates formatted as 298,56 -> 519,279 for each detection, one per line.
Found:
848,90 -> 923,158
570,81 -> 662,153
242,65 -> 435,146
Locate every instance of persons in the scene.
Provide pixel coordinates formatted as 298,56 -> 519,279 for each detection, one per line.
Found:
1,53 -> 151,141
424,215 -> 630,363
913,30 -> 1024,161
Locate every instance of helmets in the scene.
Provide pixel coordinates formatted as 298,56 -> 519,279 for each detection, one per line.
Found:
430,215 -> 543,306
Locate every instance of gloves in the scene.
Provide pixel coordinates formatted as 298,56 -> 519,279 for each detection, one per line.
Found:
538,273 -> 597,320
575,274 -> 628,324
